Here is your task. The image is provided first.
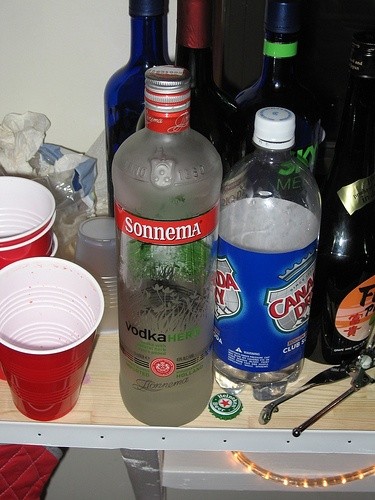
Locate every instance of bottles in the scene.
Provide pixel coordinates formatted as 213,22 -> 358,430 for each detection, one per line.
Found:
213,106 -> 322,401
304,30 -> 375,366
104,0 -> 334,218
111,65 -> 223,426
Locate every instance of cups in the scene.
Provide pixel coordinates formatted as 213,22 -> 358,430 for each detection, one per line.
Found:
74,216 -> 119,335
0,175 -> 59,271
0,256 -> 105,422
